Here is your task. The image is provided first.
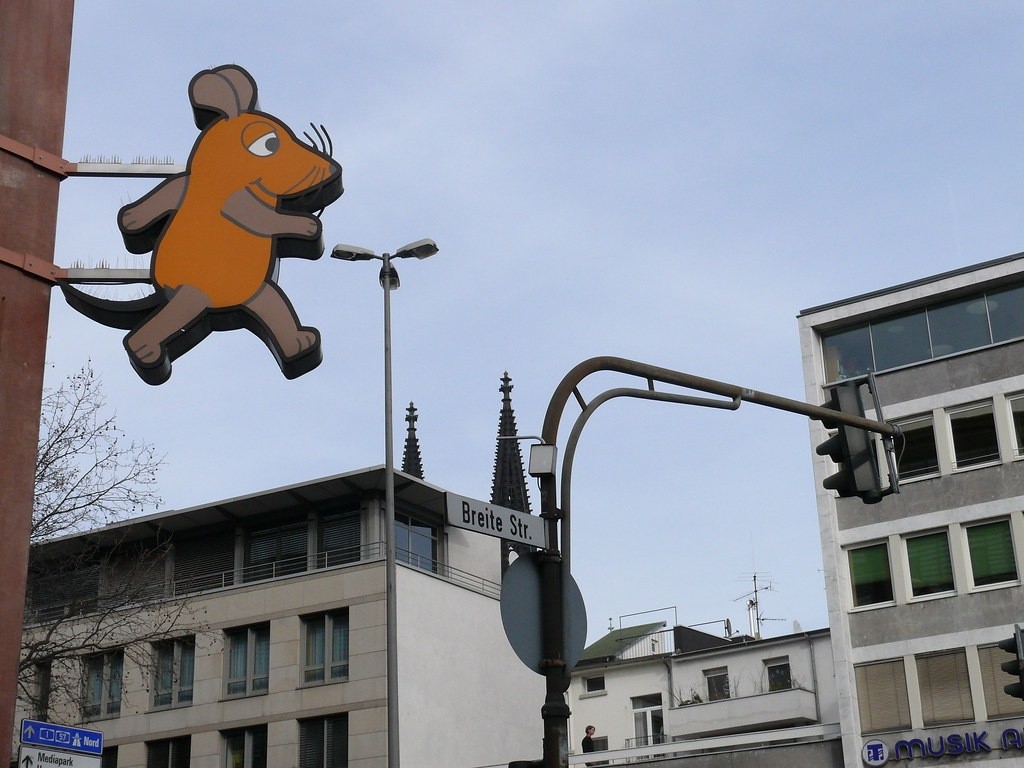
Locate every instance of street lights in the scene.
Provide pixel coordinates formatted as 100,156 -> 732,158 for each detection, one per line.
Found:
329,239 -> 440,768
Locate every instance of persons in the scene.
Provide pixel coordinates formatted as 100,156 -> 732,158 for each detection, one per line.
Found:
582,726 -> 595,766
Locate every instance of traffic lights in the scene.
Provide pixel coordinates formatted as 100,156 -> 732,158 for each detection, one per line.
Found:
997,622 -> 1024,701
810,380 -> 883,502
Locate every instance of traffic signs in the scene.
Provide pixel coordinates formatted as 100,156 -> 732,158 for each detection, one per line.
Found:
18,747 -> 101,768
20,720 -> 105,756
